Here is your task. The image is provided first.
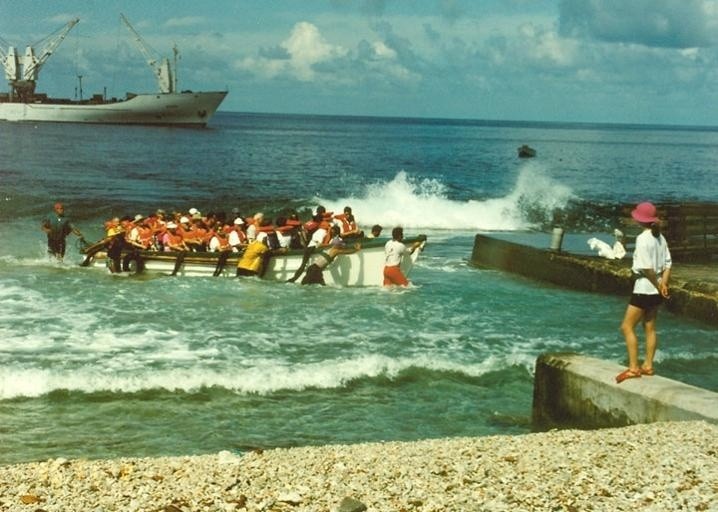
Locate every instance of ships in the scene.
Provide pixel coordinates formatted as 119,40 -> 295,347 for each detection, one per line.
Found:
0,12 -> 227,127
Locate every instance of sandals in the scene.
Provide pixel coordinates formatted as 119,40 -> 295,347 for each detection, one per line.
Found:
616,369 -> 641,382
639,365 -> 654,375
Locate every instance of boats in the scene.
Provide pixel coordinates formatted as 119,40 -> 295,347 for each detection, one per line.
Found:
80,235 -> 426,287
516,144 -> 536,157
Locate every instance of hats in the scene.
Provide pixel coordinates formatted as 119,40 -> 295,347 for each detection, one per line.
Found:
631,201 -> 662,222
149,207 -> 197,229
133,214 -> 147,224
232,207 -> 245,225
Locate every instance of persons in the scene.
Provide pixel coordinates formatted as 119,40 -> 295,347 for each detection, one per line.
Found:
102,203 -> 384,288
39,203 -> 84,265
383,227 -> 422,289
612,201 -> 673,384
